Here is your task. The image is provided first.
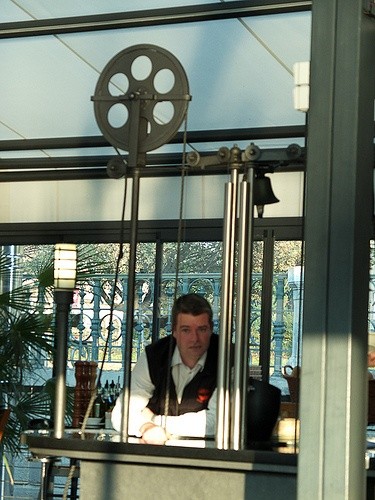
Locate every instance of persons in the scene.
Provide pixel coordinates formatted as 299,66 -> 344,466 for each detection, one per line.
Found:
111,292 -> 236,445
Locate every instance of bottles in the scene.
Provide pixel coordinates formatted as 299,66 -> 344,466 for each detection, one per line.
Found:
96,376 -> 122,406
92,394 -> 103,418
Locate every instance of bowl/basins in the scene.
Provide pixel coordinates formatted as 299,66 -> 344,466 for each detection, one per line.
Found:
85,417 -> 102,422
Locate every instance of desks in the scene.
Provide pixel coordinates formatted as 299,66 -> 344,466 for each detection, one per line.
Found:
27,436 -> 297,500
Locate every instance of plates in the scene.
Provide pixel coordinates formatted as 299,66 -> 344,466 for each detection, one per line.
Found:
79,422 -> 105,428
